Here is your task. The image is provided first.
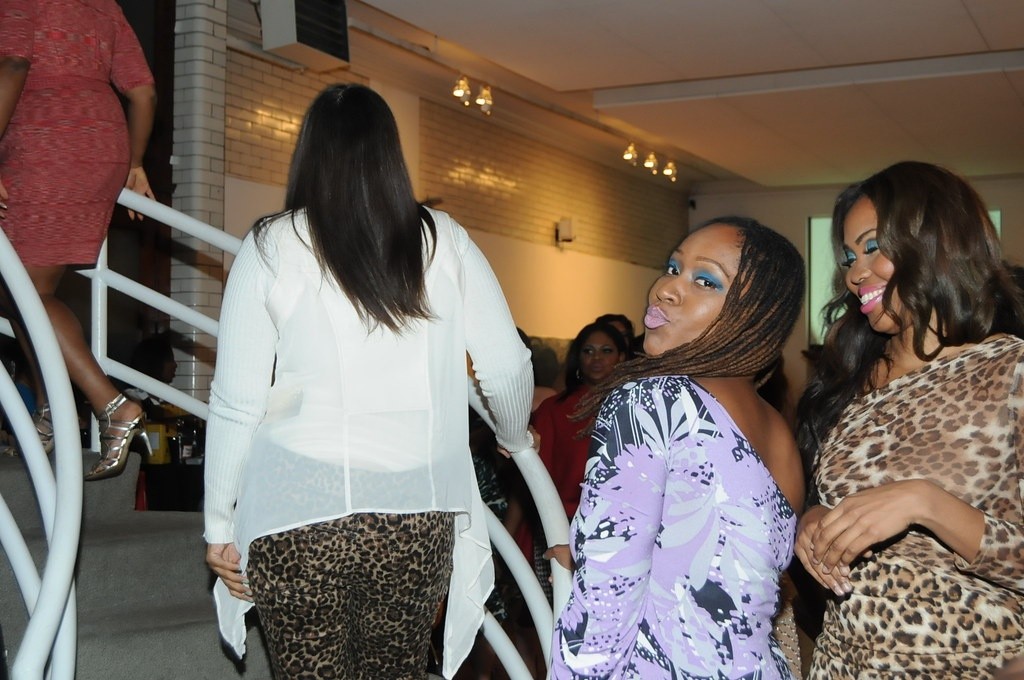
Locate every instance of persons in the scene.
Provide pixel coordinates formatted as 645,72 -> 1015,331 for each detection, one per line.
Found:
794,160 -> 1024,680
0,0 -> 156,481
5,352 -> 36,437
204,83 -> 541,678
544,216 -> 807,680
432,314 -> 635,680
124,337 -> 176,422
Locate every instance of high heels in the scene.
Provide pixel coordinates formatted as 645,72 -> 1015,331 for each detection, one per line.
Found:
83,394 -> 154,481
31,404 -> 59,454
